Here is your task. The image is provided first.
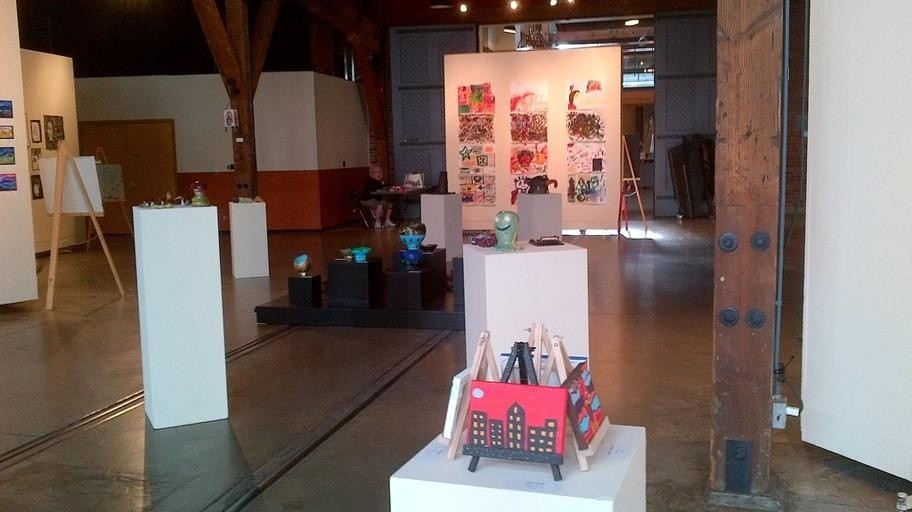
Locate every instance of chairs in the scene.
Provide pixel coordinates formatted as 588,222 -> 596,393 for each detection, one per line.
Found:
350,190 -> 375,228
431,172 -> 448,194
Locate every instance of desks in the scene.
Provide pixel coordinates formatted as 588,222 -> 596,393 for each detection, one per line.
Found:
370,185 -> 436,225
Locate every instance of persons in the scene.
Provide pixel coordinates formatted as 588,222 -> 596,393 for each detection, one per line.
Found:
359,162 -> 397,229
45,116 -> 58,149
